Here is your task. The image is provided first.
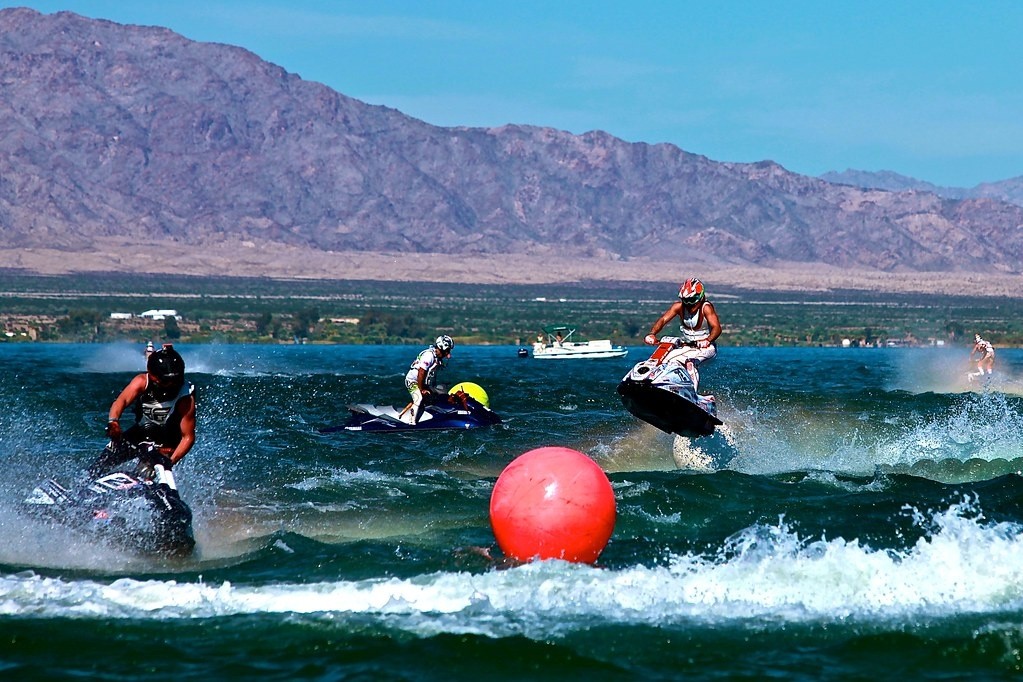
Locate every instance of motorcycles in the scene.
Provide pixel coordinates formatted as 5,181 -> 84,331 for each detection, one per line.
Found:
316,384 -> 503,432
615,336 -> 724,440
960,360 -> 1023,394
17,423 -> 196,568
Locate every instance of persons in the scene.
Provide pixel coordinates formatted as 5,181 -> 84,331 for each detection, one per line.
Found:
644,277 -> 723,394
404,333 -> 454,426
968,333 -> 995,377
75,343 -> 195,548
537,333 -> 544,344
556,332 -> 563,347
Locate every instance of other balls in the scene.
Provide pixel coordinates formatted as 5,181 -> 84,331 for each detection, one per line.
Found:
488,447 -> 614,571
446,381 -> 489,408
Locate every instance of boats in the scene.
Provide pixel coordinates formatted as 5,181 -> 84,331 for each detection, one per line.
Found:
532,324 -> 629,362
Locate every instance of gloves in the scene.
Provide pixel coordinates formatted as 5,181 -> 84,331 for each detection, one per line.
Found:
105,420 -> 120,436
644,333 -> 656,345
696,339 -> 711,348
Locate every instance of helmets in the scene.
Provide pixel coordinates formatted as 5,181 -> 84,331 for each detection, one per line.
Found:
433,334 -> 454,360
677,279 -> 705,309
147,346 -> 184,403
